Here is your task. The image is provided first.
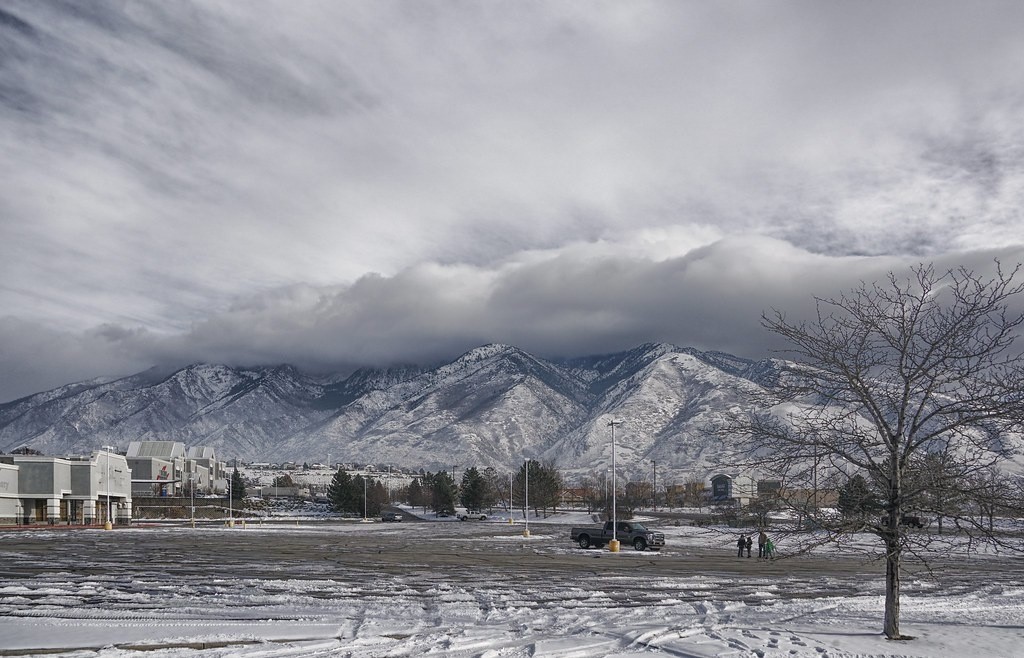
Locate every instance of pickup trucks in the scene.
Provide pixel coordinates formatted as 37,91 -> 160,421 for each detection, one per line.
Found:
880,511 -> 929,529
456,508 -> 488,521
569,520 -> 666,553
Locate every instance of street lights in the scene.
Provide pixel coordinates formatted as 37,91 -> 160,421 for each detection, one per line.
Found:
388,464 -> 391,499
608,421 -> 622,552
524,458 -> 530,537
650,459 -> 656,511
452,466 -> 458,485
188,477 -> 195,521
101,445 -> 113,529
228,471 -> 234,528
362,476 -> 368,519
509,471 -> 514,523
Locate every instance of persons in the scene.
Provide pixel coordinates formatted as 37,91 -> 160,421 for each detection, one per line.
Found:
745,537 -> 752,558
765,539 -> 774,559
737,534 -> 745,557
757,531 -> 767,558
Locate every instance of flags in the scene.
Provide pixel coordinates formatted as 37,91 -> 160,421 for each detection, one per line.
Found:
157,475 -> 160,480
167,473 -> 169,477
162,465 -> 166,470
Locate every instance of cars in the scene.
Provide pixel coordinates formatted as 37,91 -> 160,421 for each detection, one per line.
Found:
382,513 -> 402,522
